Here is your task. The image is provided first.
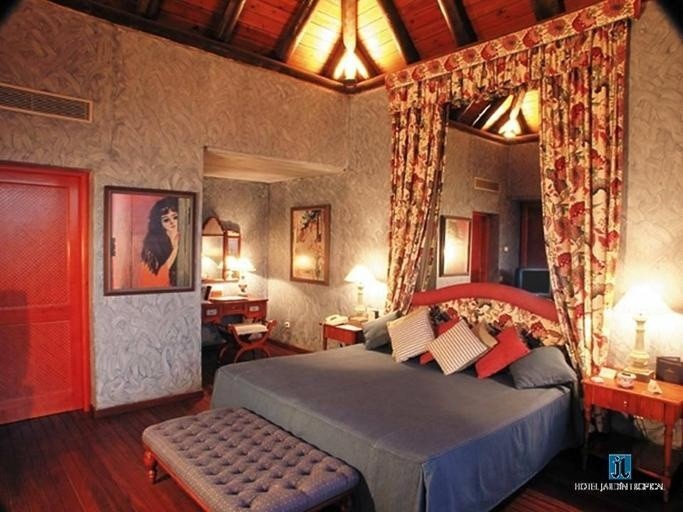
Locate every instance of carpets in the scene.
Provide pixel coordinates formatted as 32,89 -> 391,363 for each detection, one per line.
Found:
528,451 -> 682,512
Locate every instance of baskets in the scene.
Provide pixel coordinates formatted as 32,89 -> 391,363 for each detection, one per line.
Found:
656,357 -> 682,385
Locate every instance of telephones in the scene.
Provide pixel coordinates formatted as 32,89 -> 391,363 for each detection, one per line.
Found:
324,315 -> 348,325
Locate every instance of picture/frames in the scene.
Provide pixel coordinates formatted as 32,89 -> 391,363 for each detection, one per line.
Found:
438,214 -> 470,277
289,203 -> 329,286
103,185 -> 196,294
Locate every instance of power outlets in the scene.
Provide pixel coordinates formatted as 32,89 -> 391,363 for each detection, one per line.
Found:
284,321 -> 290,328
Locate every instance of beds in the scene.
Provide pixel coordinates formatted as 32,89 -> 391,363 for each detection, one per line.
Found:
210,279 -> 583,511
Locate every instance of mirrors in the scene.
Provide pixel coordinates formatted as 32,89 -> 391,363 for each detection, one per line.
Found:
431,84 -> 558,305
200,234 -> 241,282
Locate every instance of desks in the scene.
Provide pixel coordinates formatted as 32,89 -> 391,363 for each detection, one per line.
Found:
200,297 -> 269,356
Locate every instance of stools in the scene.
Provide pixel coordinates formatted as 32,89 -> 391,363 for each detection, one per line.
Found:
215,316 -> 277,360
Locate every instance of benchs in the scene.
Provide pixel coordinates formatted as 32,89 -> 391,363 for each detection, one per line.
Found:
142,405 -> 361,512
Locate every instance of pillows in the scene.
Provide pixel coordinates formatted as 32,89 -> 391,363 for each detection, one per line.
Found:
359,310 -> 578,388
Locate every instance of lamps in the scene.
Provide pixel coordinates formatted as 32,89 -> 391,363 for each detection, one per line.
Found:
344,265 -> 373,313
231,257 -> 255,296
615,287 -> 668,381
217,254 -> 237,282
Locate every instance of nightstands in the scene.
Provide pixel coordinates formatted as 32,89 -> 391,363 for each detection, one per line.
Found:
580,366 -> 682,504
318,319 -> 362,351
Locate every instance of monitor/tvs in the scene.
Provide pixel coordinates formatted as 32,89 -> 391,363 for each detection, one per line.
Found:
516,267 -> 552,299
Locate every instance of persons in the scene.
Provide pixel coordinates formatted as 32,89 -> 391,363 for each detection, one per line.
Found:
140,197 -> 180,286
